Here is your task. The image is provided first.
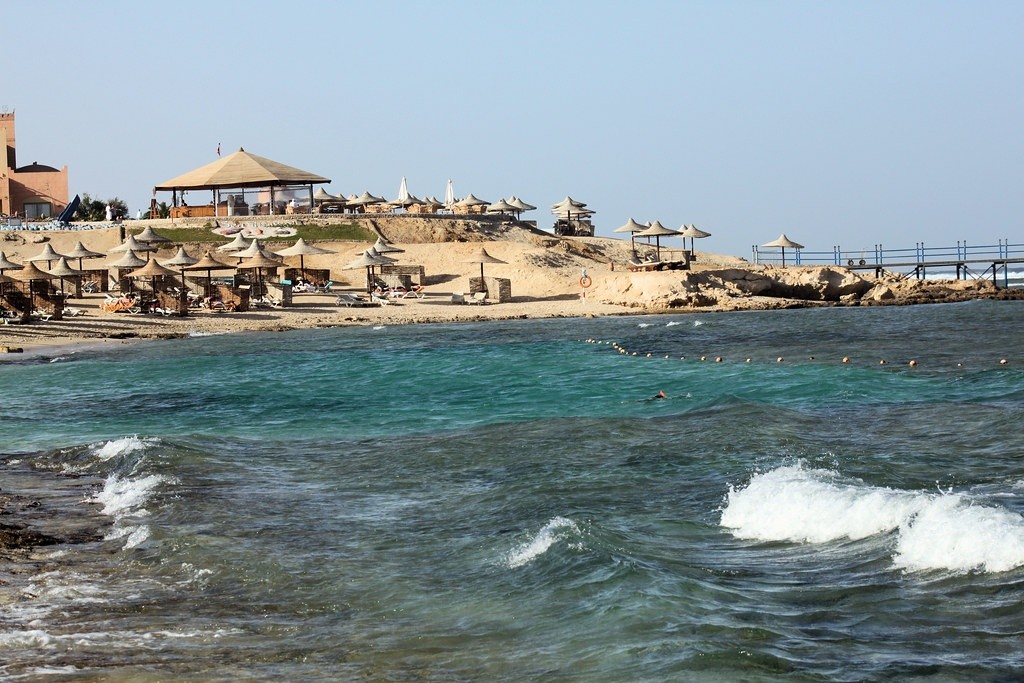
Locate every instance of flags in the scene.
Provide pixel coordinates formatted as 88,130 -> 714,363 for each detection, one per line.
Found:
217,146 -> 221,157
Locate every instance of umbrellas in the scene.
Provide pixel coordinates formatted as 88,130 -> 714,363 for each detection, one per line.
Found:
1,226 -> 406,319
309,188 -> 712,261
761,235 -> 805,265
461,248 -> 509,294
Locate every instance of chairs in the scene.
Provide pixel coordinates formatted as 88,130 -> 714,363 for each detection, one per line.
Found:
625,254 -> 684,272
0,274 -> 488,325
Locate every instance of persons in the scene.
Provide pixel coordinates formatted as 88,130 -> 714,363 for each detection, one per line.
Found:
106,204 -> 112,221
289,199 -> 299,208
137,208 -> 143,220
110,205 -> 122,221
